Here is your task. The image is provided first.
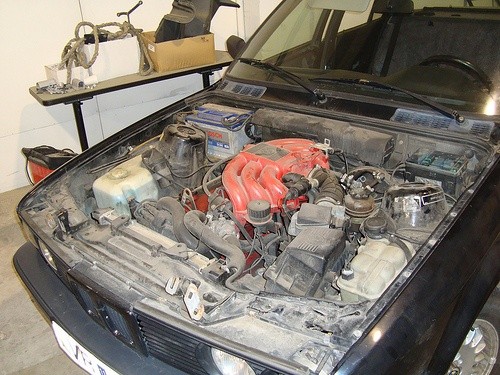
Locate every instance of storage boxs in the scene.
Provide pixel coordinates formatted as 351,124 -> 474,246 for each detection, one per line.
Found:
141,30 -> 216,72
82,33 -> 140,83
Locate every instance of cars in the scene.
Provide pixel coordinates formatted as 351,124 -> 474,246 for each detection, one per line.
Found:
12,0 -> 500,375
